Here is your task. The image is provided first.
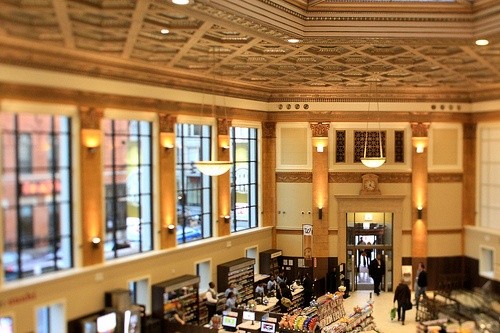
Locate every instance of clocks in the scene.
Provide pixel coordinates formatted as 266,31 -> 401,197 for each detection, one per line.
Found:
364,180 -> 375,192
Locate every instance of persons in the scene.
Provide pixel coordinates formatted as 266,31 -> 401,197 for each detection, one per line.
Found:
205,281 -> 220,323
224,281 -> 241,310
357,237 -> 373,267
275,282 -> 293,314
368,253 -> 385,296
255,271 -> 287,299
394,279 -> 411,326
302,274 -> 313,307
415,263 -> 428,310
170,302 -> 190,333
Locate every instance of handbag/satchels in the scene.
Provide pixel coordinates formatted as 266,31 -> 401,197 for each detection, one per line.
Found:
406,301 -> 412,310
391,303 -> 396,320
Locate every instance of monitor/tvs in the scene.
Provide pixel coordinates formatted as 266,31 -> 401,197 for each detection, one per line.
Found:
260,321 -> 276,333
222,315 -> 238,331
242,310 -> 256,321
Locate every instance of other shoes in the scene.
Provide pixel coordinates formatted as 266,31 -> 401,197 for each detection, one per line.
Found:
374,289 -> 380,295
402,318 -> 404,325
398,316 -> 400,321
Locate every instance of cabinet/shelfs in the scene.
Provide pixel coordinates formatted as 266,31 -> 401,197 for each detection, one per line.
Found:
260,248 -> 281,283
217,257 -> 256,305
154,275 -> 201,325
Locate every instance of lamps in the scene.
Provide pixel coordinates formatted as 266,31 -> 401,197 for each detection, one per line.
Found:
417,208 -> 423,219
417,145 -> 425,154
361,74 -> 387,168
318,208 -> 323,219
224,215 -> 229,223
196,46 -> 232,176
317,145 -> 324,153
